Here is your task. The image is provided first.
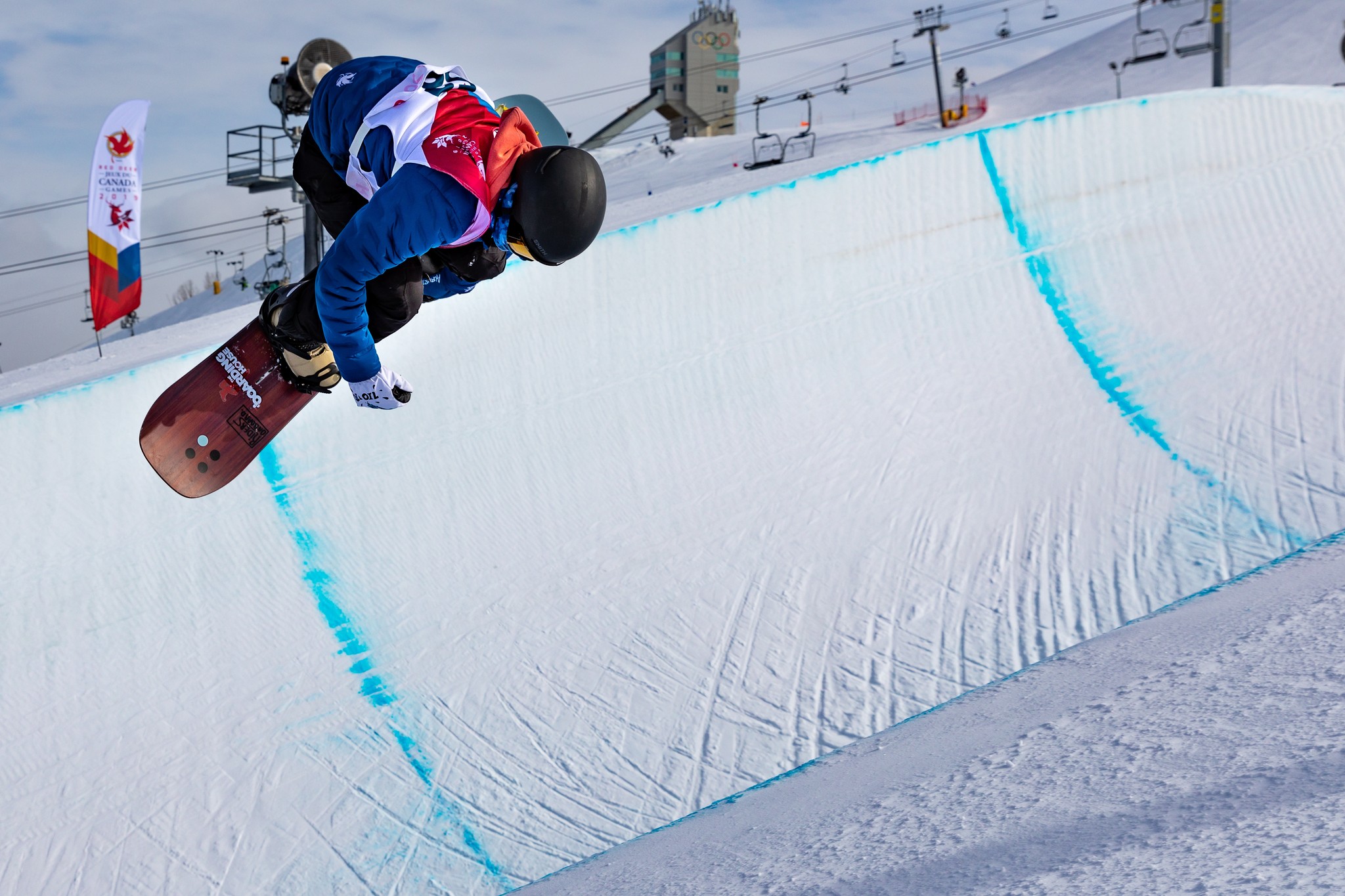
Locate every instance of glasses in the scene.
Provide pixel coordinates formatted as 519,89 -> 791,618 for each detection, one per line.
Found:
505,233 -> 537,263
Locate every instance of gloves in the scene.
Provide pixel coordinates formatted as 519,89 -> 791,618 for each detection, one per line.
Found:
349,365 -> 412,410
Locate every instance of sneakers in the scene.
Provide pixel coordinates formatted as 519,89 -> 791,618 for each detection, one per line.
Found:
271,306 -> 341,386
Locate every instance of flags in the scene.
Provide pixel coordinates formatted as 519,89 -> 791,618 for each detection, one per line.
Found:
88,98 -> 149,331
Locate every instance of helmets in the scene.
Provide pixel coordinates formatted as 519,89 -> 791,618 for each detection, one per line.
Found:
511,146 -> 606,266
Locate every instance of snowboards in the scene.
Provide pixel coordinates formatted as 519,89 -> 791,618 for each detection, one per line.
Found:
139,94 -> 568,499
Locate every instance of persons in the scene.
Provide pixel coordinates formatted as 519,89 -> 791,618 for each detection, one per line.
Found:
272,56 -> 607,410
240,277 -> 248,291
